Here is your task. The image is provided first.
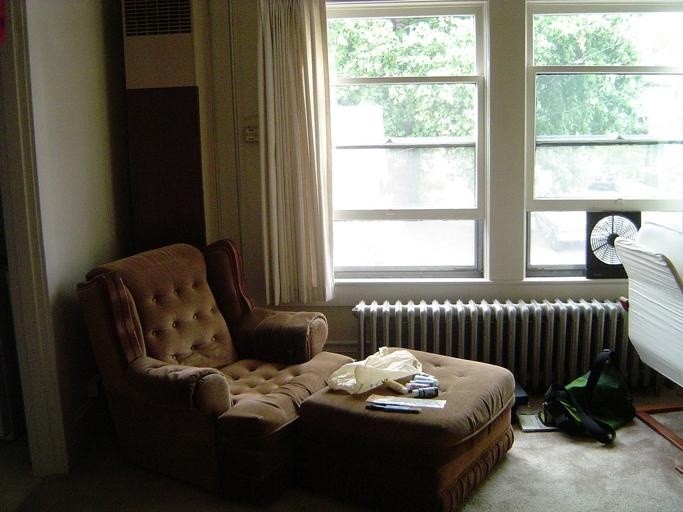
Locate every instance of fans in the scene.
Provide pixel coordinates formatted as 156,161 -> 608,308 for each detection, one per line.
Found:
584,210 -> 640,279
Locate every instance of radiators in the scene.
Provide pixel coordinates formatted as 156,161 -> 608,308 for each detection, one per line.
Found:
349,295 -> 664,401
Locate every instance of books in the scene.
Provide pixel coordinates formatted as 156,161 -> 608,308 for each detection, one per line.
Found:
516,413 -> 560,433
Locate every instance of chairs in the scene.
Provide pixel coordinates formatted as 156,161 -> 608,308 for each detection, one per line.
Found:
612,236 -> 682,449
74,237 -> 355,510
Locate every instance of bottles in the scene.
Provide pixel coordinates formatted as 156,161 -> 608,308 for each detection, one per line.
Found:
387,368 -> 439,398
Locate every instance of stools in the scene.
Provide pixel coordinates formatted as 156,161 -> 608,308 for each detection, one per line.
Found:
297,346 -> 517,509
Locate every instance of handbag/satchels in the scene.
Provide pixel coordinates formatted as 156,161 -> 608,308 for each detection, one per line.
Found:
540,349 -> 636,449
